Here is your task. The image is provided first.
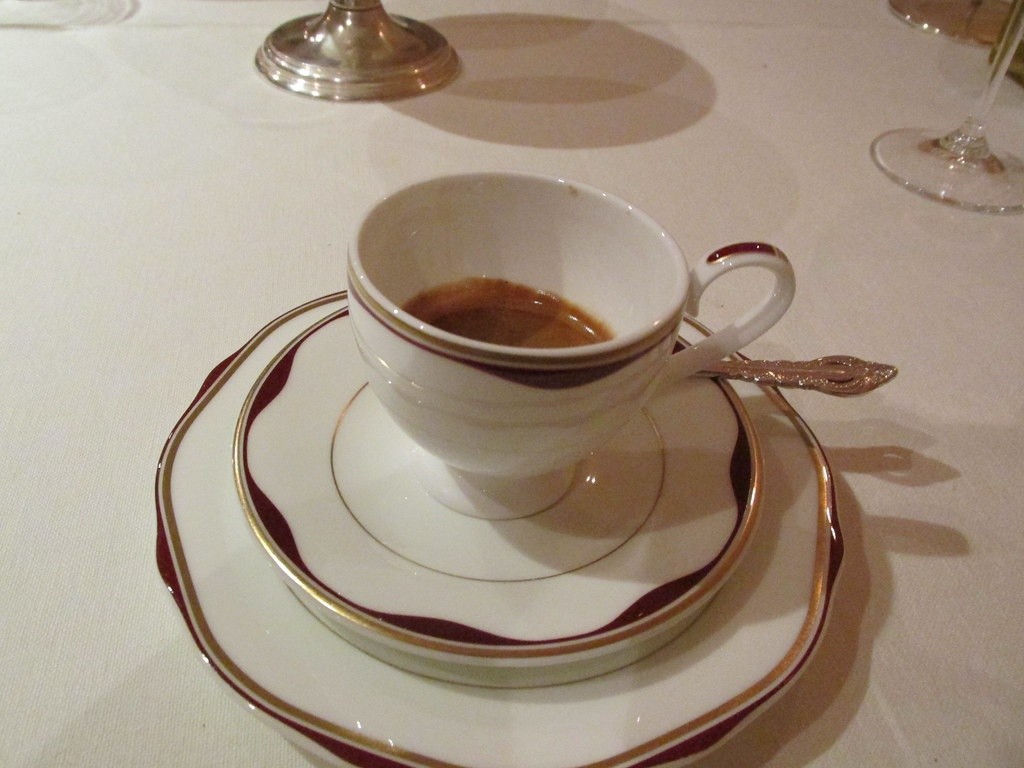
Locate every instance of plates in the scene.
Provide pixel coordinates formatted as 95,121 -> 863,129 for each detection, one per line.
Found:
153,291 -> 845,767
232,304 -> 765,668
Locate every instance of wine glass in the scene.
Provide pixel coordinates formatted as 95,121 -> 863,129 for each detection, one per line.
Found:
869,1 -> 1023,213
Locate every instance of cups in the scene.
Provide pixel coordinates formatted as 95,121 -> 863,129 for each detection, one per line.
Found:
346,171 -> 795,478
